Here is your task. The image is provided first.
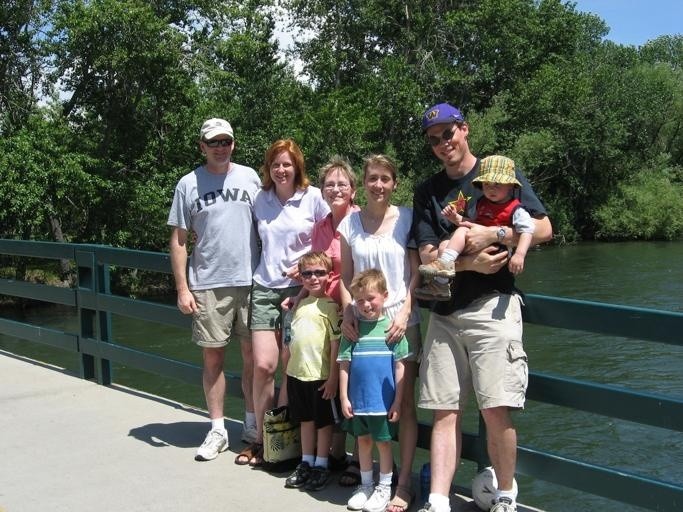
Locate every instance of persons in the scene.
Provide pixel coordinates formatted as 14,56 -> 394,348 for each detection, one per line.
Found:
280,156 -> 365,486
336,270 -> 412,512
165,117 -> 263,461
285,251 -> 343,493
191,140 -> 333,466
412,103 -> 553,512
413,156 -> 534,302
336,155 -> 422,512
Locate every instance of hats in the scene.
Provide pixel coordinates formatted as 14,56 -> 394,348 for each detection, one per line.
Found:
470,154 -> 523,193
422,102 -> 464,129
200,117 -> 235,140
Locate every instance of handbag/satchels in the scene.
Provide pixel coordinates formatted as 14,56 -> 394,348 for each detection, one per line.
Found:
262,407 -> 301,465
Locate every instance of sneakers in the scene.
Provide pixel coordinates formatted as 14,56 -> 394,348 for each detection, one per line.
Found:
417,501 -> 454,512
303,466 -> 334,491
240,422 -> 258,443
413,280 -> 454,302
195,428 -> 231,461
285,461 -> 310,488
363,484 -> 394,512
417,258 -> 457,278
347,482 -> 376,511
488,498 -> 520,511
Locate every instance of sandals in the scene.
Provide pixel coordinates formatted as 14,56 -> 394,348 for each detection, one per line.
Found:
338,461 -> 362,488
387,484 -> 417,512
327,451 -> 349,471
234,444 -> 260,465
249,447 -> 266,466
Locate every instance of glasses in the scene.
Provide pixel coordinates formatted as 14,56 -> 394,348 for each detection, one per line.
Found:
201,139 -> 234,147
299,269 -> 329,278
428,124 -> 462,147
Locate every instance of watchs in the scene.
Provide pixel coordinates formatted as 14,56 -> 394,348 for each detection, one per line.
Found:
497,226 -> 506,242
